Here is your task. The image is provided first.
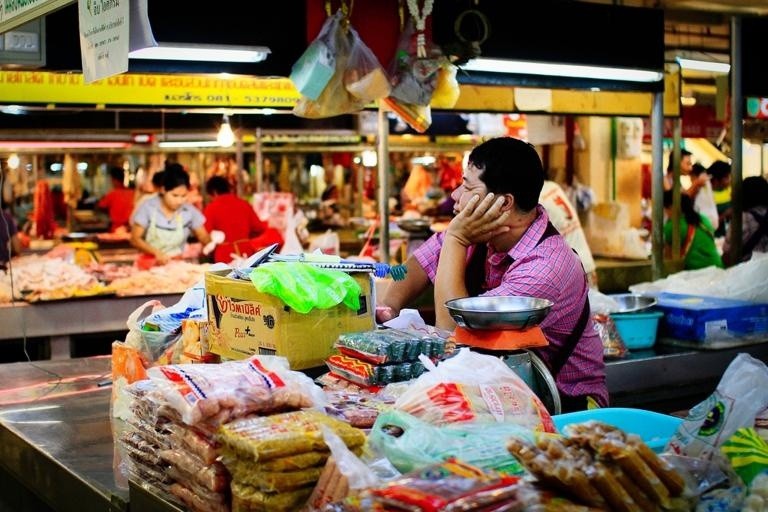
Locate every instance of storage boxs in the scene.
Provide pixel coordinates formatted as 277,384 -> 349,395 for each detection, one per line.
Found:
198,267 -> 374,372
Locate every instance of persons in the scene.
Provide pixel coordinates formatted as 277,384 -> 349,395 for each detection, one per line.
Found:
131,165 -> 209,262
376,135 -> 611,413
663,148 -> 768,269
201,174 -> 267,243
95,166 -> 134,234
540,175 -> 598,290
322,166 -> 339,212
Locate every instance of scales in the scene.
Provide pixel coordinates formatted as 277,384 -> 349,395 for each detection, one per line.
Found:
397,219 -> 432,261
60,232 -> 99,271
443,295 -> 561,417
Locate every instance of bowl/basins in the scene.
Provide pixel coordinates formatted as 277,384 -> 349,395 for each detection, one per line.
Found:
608,311 -> 666,351
607,293 -> 658,312
